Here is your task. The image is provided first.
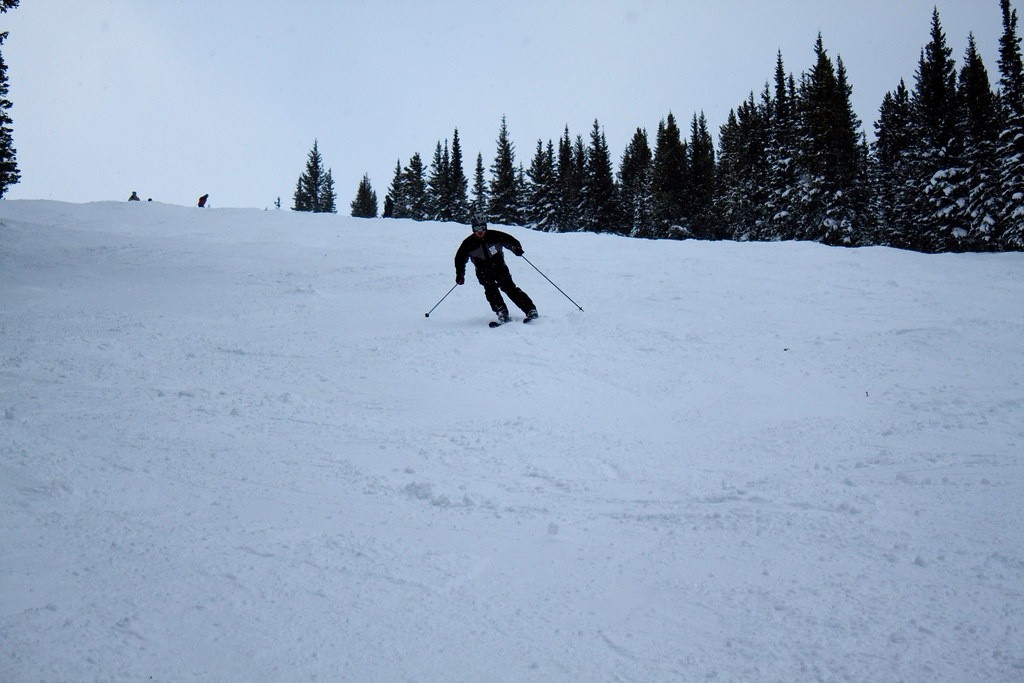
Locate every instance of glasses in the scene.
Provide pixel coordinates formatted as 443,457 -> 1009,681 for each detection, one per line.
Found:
472,223 -> 487,232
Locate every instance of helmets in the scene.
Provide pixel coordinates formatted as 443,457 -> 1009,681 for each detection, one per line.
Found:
471,213 -> 487,224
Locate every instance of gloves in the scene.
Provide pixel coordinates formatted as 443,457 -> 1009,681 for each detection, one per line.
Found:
456,276 -> 465,285
514,247 -> 524,257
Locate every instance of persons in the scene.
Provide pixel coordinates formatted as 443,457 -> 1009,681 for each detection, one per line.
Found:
455,212 -> 537,323
128,191 -> 140,202
198,193 -> 209,208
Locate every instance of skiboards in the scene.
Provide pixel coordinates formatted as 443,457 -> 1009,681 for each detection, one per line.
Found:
523,316 -> 534,324
489,321 -> 506,328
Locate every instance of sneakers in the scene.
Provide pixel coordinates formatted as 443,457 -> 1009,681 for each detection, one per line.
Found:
527,310 -> 538,319
498,311 -> 509,324
